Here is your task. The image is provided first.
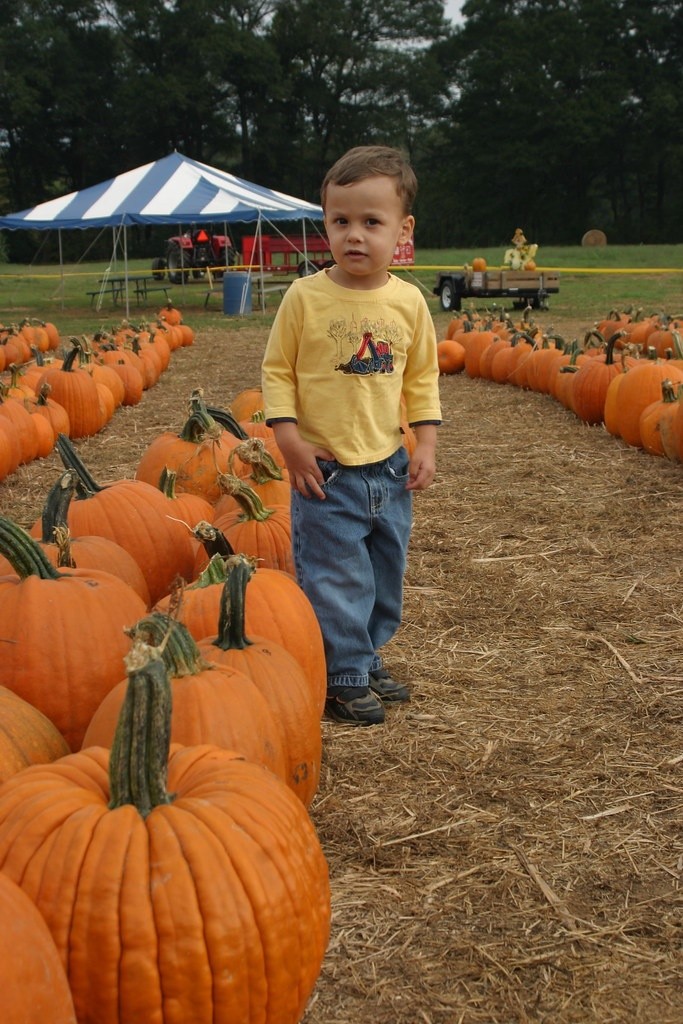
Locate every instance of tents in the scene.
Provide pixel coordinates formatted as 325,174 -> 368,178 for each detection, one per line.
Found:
0,147 -> 324,317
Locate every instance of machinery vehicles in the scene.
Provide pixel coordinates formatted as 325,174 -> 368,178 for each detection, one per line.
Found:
151,223 -> 234,284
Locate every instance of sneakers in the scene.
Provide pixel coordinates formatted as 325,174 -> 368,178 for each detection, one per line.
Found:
324,686 -> 385,725
368,670 -> 408,701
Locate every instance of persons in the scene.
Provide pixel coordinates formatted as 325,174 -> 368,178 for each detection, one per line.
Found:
262,145 -> 442,727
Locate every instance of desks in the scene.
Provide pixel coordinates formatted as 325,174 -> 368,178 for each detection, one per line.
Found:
99,275 -> 158,305
215,271 -> 272,305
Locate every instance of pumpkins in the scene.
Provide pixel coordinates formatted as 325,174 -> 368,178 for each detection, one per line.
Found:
524,260 -> 536,270
437,302 -> 683,467
473,256 -> 486,272
0,299 -> 336,1024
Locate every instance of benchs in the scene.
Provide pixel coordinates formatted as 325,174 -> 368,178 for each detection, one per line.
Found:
254,286 -> 288,305
133,285 -> 172,306
198,287 -> 223,308
87,288 -> 125,308
